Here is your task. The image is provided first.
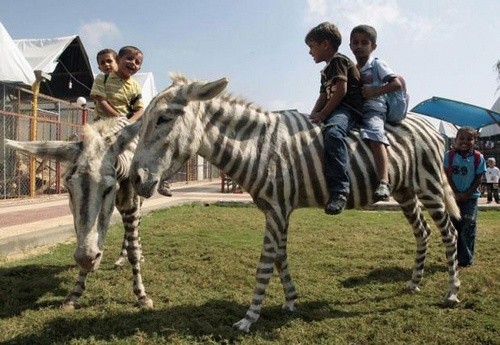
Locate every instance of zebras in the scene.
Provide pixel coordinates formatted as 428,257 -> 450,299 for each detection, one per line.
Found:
6,112 -> 153,311
129,73 -> 463,335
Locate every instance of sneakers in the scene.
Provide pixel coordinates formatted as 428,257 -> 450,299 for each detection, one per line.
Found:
157,180 -> 172,196
372,179 -> 392,201
325,192 -> 349,215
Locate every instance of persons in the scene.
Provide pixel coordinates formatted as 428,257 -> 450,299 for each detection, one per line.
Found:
349,25 -> 403,203
91,45 -> 145,124
96,48 -> 174,198
305,21 -> 362,216
444,127 -> 488,266
486,157 -> 500,205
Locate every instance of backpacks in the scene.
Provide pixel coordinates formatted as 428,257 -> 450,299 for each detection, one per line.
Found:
371,58 -> 408,124
446,150 -> 481,182
